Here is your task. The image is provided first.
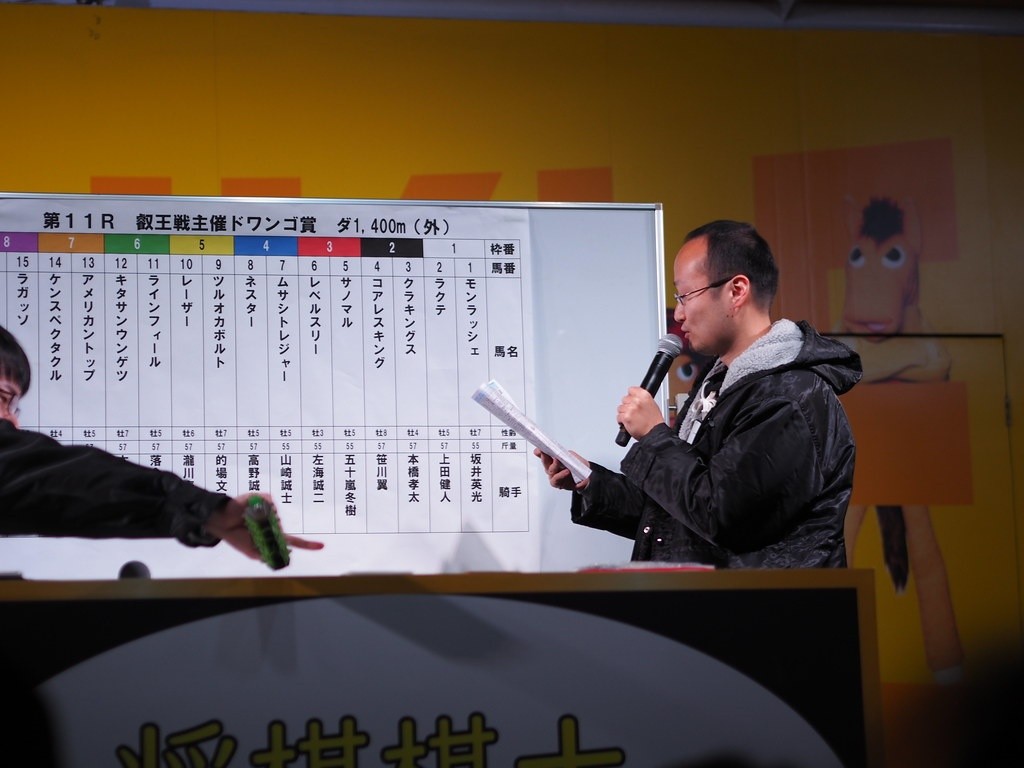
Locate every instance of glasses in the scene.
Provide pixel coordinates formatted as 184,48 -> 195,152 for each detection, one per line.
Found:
0,388 -> 21,419
674,272 -> 753,306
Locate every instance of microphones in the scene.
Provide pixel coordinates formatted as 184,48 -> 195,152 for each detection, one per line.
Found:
616,333 -> 684,448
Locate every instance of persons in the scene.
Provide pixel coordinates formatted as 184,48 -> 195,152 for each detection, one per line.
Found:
1,328 -> 325,559
535,220 -> 864,569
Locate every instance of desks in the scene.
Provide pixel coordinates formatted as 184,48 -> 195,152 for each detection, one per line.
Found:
0,568 -> 888,768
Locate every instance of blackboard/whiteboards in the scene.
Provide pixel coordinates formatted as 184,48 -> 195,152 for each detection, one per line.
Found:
0,191 -> 668,579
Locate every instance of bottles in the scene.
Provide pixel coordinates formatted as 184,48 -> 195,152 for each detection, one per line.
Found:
244,496 -> 289,569
